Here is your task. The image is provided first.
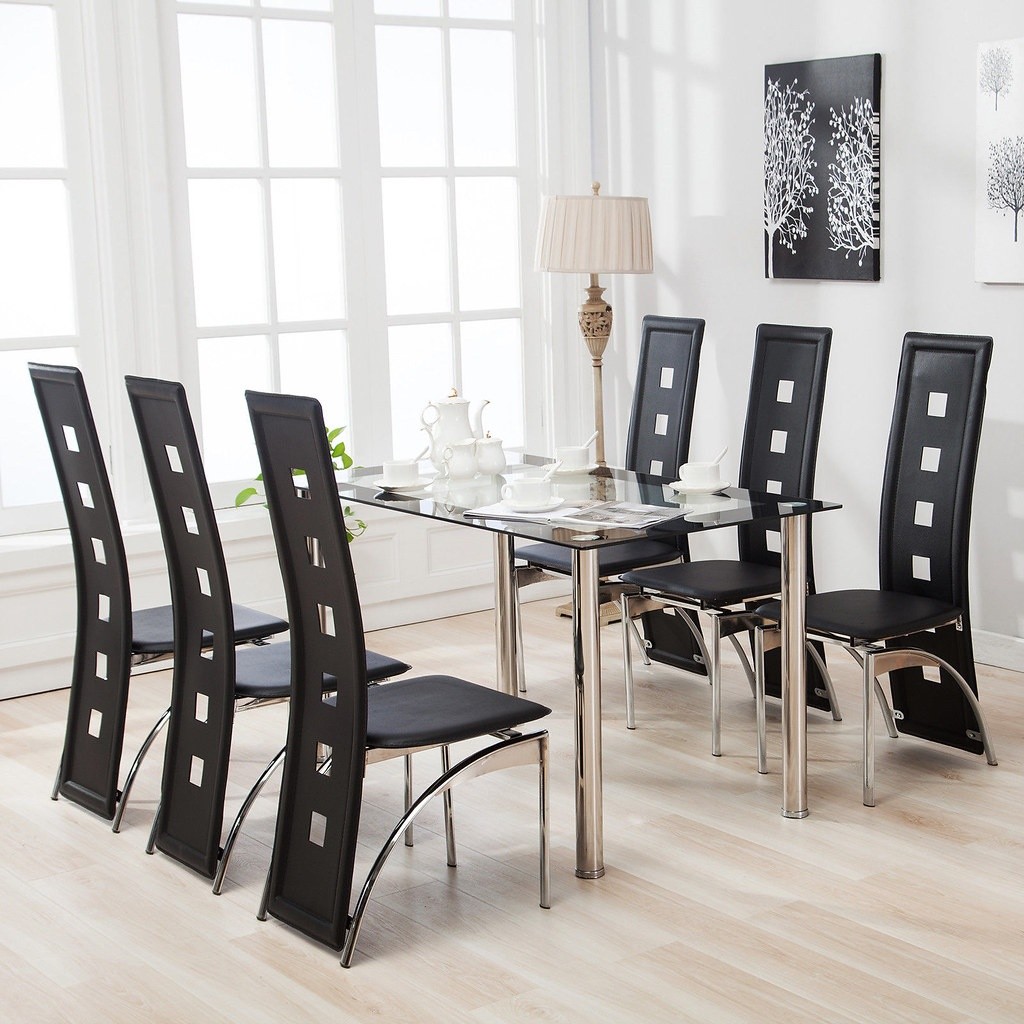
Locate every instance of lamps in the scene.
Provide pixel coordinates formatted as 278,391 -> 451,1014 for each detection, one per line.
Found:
538,181 -> 654,466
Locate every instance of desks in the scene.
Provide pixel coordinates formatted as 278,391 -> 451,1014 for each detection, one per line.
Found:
333,451 -> 845,887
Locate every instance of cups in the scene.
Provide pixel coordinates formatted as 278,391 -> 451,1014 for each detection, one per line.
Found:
501,478 -> 550,502
383,462 -> 418,484
557,447 -> 589,468
476,438 -> 506,475
679,462 -> 720,486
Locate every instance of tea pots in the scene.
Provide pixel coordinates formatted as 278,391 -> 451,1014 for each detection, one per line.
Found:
420,389 -> 489,474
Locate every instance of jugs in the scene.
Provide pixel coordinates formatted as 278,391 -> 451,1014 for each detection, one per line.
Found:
440,438 -> 475,480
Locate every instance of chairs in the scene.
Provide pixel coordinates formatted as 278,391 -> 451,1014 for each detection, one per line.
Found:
242,386 -> 554,969
624,323 -> 844,758
123,373 -> 412,897
27,359 -> 291,835
508,314 -> 713,694
749,332 -> 1000,808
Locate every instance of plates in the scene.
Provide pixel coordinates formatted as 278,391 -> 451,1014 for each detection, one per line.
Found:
670,482 -> 730,493
374,479 -> 434,491
543,464 -> 600,474
511,497 -> 566,513
